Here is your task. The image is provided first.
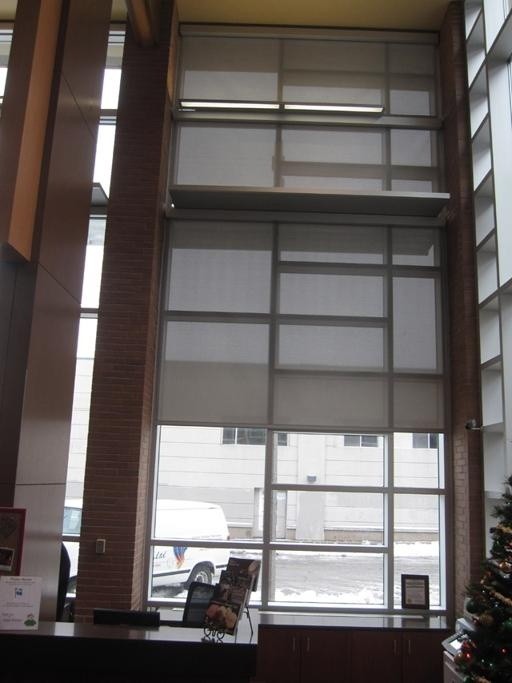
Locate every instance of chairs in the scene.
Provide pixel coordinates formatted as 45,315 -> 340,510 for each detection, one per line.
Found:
159,581 -> 218,628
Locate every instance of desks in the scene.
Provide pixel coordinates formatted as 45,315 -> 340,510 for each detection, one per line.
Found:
1,621 -> 257,682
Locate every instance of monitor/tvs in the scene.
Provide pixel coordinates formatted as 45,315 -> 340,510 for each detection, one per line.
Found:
93,608 -> 159,627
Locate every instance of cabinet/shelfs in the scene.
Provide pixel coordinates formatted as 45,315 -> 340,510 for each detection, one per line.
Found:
351,613 -> 457,682
257,612 -> 352,682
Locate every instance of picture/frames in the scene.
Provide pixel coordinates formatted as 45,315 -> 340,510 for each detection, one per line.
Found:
400,573 -> 430,610
1,506 -> 28,577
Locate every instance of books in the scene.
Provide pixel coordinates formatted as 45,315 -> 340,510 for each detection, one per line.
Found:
203,557 -> 262,640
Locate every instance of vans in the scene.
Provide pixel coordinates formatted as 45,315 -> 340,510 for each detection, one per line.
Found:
59,496 -> 231,595
